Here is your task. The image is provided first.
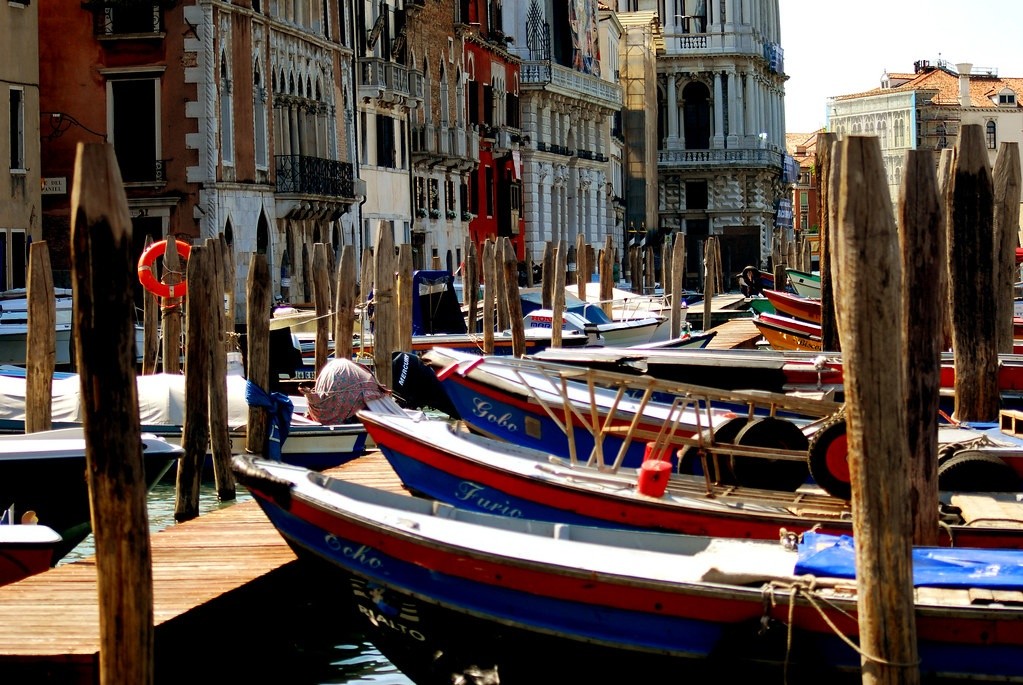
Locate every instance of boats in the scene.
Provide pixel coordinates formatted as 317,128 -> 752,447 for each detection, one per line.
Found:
0,424 -> 186,566
1,500 -> 65,585
301,355 -> 1023,555
0,264 -> 1023,494
225,452 -> 1023,685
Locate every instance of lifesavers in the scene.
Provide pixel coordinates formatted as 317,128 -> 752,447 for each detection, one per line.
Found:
702,616 -> 829,685
742,265 -> 760,286
680,446 -> 722,478
938,451 -> 1021,492
136,238 -> 191,299
